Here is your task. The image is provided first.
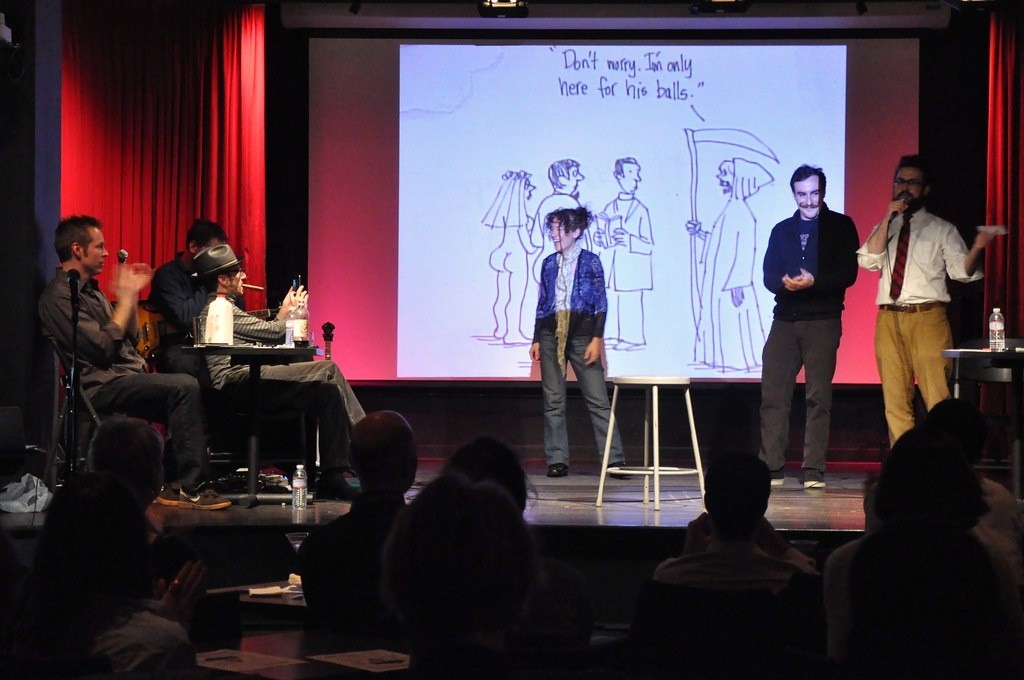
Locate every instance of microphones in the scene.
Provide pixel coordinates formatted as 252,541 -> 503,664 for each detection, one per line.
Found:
117,250 -> 128,263
888,195 -> 909,224
67,269 -> 81,310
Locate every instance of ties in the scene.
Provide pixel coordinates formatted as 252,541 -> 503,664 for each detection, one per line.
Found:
889,214 -> 913,302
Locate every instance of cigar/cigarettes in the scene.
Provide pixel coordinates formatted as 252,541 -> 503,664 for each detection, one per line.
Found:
242,284 -> 264,291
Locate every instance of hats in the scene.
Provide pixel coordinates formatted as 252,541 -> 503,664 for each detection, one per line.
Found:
190,244 -> 246,277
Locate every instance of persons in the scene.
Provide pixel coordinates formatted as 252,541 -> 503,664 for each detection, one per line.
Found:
823,426 -> 1024,662
652,450 -> 821,589
148,217 -> 244,376
856,154 -> 999,448
444,437 -> 594,647
190,244 -> 367,500
0,415 -> 241,680
925,398 -> 1024,585
759,164 -> 859,488
297,411 -> 418,633
386,473 -> 537,680
38,216 -> 232,511
529,205 -> 632,479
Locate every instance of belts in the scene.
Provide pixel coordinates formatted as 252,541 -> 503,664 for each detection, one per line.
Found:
879,303 -> 941,314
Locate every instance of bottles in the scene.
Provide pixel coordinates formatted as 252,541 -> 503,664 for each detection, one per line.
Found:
286,301 -> 309,347
988,306 -> 1006,352
292,464 -> 308,511
204,293 -> 237,345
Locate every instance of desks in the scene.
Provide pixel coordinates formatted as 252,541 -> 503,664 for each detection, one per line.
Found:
180,346 -> 317,507
943,348 -> 1023,501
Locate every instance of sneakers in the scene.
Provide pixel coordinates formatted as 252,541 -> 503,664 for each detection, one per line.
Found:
156,482 -> 181,506
179,485 -> 231,512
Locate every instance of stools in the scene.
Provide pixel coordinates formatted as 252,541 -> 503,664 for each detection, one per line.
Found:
595,377 -> 706,510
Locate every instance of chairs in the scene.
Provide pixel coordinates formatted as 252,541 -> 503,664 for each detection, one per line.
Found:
47,339 -> 104,493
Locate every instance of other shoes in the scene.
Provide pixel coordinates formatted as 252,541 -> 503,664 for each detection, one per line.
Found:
611,463 -> 628,478
546,461 -> 567,475
315,473 -> 361,499
769,470 -> 783,487
803,467 -> 825,489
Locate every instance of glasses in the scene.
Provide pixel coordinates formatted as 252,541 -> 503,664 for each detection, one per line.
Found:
893,177 -> 926,186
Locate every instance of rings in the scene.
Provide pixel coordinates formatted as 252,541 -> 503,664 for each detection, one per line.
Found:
173,580 -> 182,584
169,586 -> 176,590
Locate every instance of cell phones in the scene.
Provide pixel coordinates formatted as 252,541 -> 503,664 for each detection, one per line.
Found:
293,275 -> 301,297
